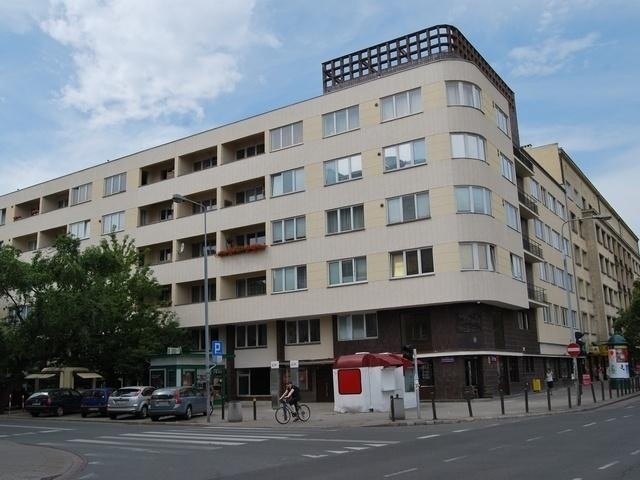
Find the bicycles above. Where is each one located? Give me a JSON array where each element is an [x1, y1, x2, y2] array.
[[274, 399, 312, 425]]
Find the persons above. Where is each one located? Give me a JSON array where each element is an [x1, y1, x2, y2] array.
[[279, 381, 301, 421], [544, 368, 555, 395]]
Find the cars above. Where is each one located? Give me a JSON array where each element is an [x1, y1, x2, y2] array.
[[20, 385, 217, 420]]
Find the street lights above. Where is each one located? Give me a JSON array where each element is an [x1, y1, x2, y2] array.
[[172, 192, 215, 425], [559, 214, 615, 341]]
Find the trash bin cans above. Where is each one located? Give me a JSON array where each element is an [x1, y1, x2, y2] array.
[[228, 401, 242, 422], [389, 398, 405, 420]]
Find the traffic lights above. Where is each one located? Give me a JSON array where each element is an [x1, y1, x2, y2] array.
[[402, 343, 414, 361], [574, 330, 585, 348]]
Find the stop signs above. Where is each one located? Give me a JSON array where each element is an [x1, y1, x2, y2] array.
[[566, 342, 582, 358]]
